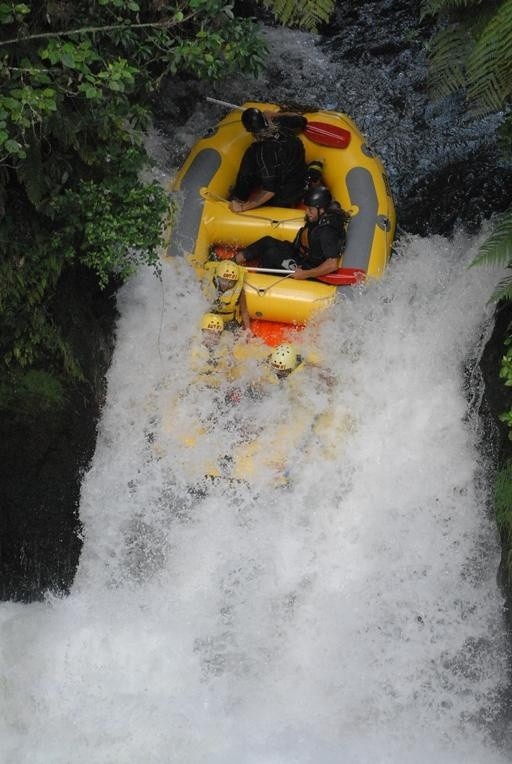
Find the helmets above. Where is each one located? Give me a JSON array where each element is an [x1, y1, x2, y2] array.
[[241, 107, 269, 132], [303, 186, 332, 209], [270, 344, 298, 372], [215, 260, 240, 281], [199, 313, 224, 331]]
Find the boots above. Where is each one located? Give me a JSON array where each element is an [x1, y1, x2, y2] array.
[[209, 250, 236, 262]]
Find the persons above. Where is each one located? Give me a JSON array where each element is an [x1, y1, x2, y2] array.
[[228, 105, 308, 212], [200, 259, 253, 339], [207, 185, 346, 280], [178, 313, 234, 412], [248, 342, 307, 402]]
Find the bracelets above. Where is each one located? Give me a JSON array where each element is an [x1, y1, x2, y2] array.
[[239, 201, 243, 212]]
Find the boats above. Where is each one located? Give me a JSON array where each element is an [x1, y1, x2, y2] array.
[[155, 98, 398, 494]]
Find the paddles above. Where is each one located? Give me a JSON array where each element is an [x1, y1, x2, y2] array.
[[199, 94, 350, 149], [205, 259, 365, 286]]
